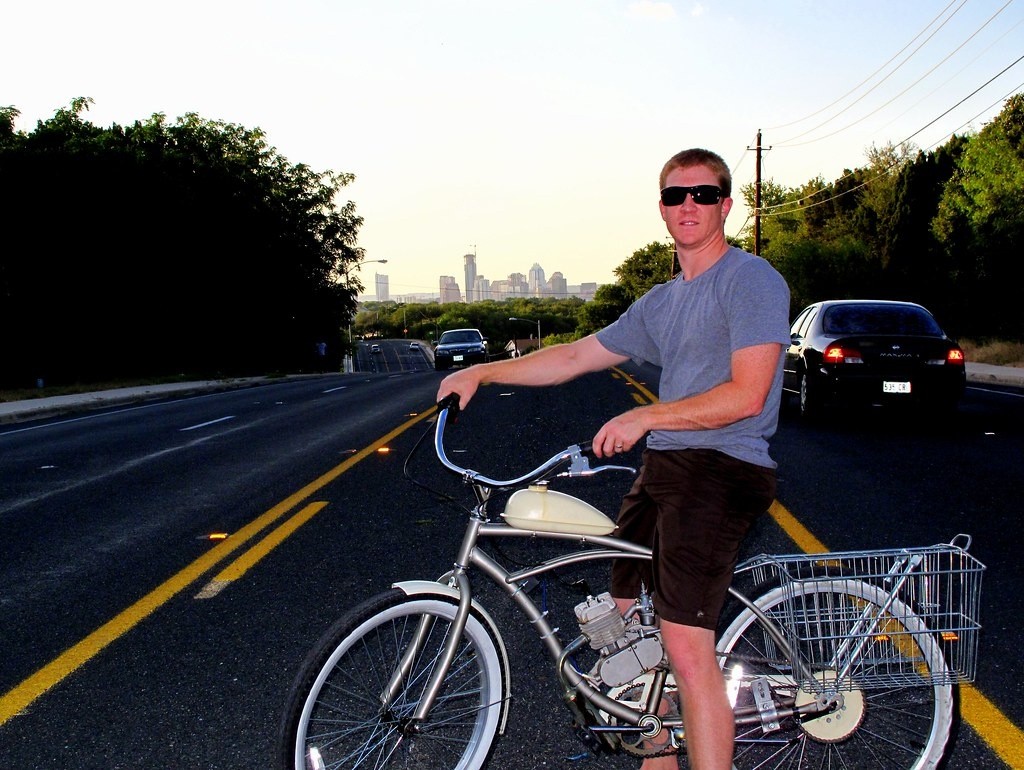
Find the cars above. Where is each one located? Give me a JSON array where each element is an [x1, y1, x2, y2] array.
[[781, 299, 967, 422], [370, 344, 381, 354], [433, 328, 490, 371], [410, 342, 419, 350]]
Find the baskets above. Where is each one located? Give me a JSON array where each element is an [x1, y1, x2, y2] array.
[[734, 531, 986, 694]]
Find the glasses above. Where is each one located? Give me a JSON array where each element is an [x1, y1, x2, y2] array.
[[661, 185, 721, 206]]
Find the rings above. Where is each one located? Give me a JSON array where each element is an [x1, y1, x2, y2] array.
[[614, 444, 622, 448]]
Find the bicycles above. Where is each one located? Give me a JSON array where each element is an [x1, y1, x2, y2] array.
[[278, 386, 987, 770]]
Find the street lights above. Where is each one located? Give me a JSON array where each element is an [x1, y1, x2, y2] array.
[[509, 317, 541, 351], [346, 259, 388, 355]]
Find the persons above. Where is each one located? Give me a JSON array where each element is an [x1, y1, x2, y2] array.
[[436, 149, 789, 770]]
